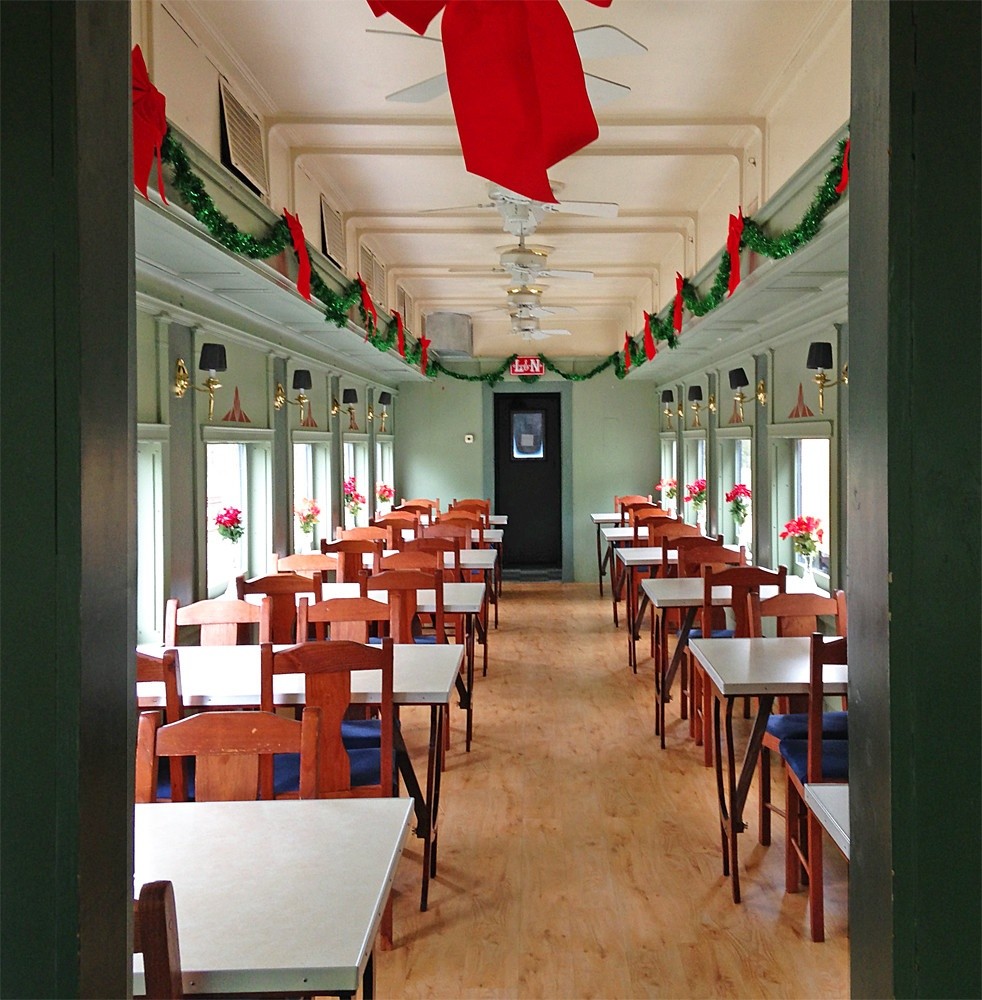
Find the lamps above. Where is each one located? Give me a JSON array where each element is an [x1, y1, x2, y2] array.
[[174, 342, 392, 420], [660, 342, 848, 428]]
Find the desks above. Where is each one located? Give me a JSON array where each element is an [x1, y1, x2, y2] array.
[[590, 513, 848, 904], [133, 516, 509, 1000]]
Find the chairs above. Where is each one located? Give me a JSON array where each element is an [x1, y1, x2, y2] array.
[[614, 494, 848, 944], [130, 497, 490, 1000]]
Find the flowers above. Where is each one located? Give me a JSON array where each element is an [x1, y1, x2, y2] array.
[[682, 479, 706, 509], [375, 483, 395, 503], [780, 515, 823, 555], [214, 506, 244, 544], [343, 477, 366, 514], [726, 484, 752, 527], [666, 480, 677, 498], [292, 498, 320, 533]]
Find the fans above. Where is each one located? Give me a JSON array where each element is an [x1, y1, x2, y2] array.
[[360, 24, 647, 340]]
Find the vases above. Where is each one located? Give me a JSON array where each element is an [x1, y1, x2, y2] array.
[[800, 554, 817, 593], [737, 525, 749, 557], [224, 545, 241, 599], [696, 510, 706, 535], [301, 535, 311, 555]]
